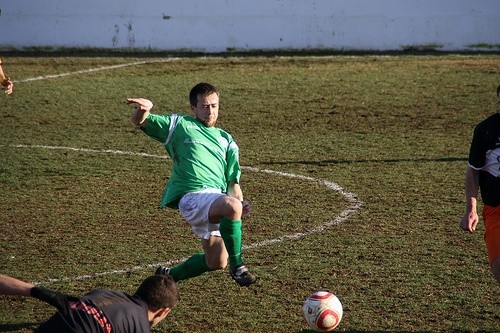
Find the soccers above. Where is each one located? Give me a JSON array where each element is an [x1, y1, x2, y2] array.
[[304, 291, 343, 332]]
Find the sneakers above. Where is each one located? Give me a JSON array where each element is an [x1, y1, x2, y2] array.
[[229, 265, 257, 287], [155, 266, 170, 275]]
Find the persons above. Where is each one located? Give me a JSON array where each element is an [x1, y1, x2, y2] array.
[[0, 59, 13, 95], [459, 82, 500, 288], [126, 82, 257, 287], [0, 271, 178, 333]]
